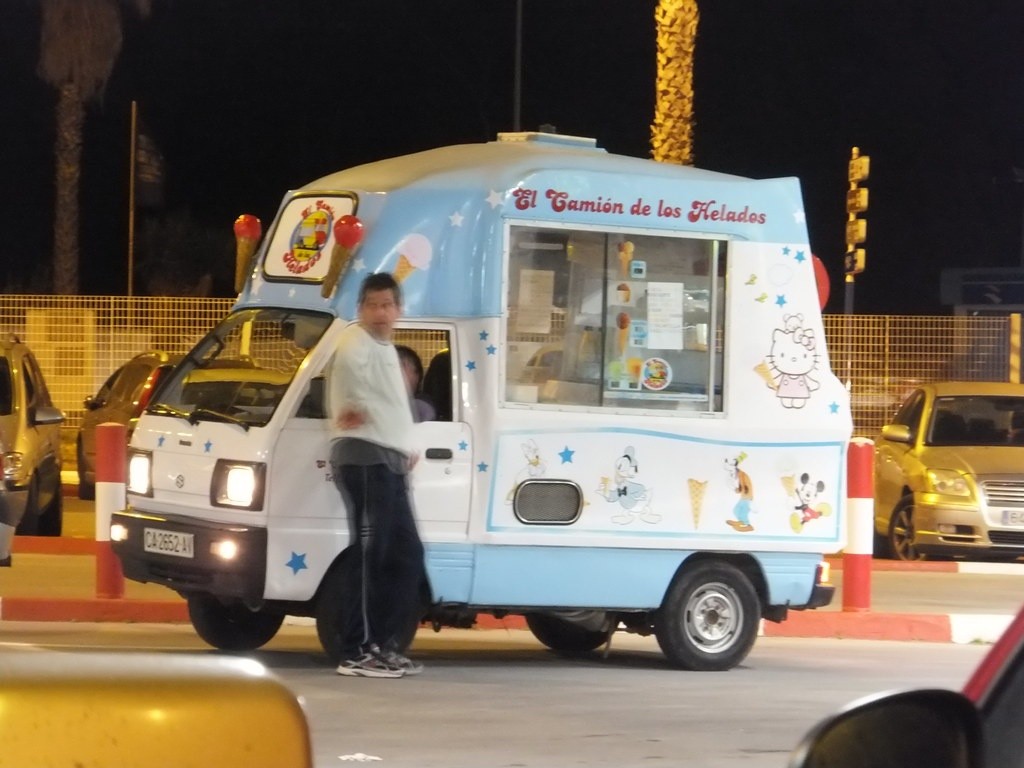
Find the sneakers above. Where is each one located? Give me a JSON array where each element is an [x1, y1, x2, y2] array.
[[336, 641, 405, 678], [381, 646, 424, 675]]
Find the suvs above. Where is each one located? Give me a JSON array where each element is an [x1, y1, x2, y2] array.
[[0, 333, 67, 537], [76, 350, 262, 500]]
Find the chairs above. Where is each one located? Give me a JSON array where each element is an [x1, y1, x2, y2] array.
[[934, 414, 966, 434], [422, 330, 453, 421]]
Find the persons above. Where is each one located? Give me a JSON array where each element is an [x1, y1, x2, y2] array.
[[324, 272, 430, 678], [393, 346, 437, 424]]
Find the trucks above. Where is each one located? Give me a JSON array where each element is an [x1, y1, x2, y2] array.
[[108, 131, 853, 672]]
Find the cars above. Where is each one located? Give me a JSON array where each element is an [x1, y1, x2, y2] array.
[[874, 381, 1024, 561], [789, 605, 1024, 768]]
